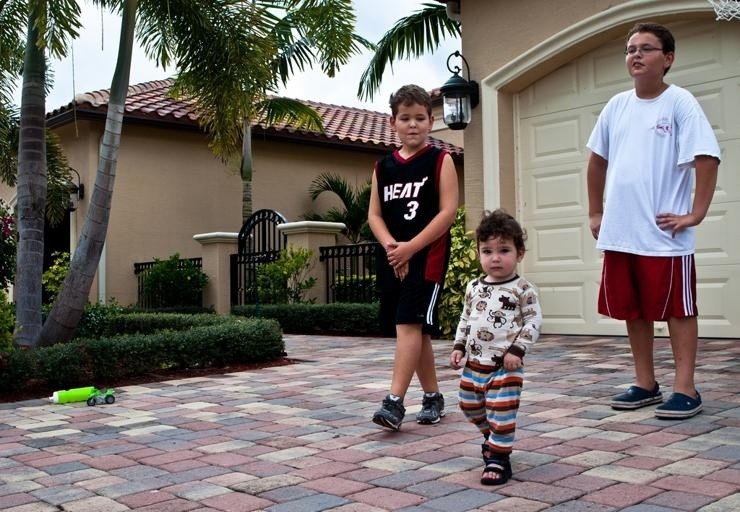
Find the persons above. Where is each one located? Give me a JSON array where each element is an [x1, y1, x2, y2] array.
[[365, 84, 461, 435], [582, 21, 725, 420], [449, 209, 544, 486]]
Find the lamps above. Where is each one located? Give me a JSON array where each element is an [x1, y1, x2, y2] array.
[[62, 167, 83, 212], [441, 52, 480, 130]]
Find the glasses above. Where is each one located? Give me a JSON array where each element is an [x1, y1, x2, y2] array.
[[624, 47, 663, 55]]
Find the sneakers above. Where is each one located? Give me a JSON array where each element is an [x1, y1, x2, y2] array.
[[416, 391, 445, 425], [610, 380, 662, 410], [656, 388, 703, 420], [374, 395, 406, 432]]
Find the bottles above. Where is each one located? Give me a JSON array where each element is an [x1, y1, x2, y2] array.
[[48, 386, 94, 403]]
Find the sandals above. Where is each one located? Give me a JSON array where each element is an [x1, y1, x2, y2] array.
[[481, 434, 512, 485]]
